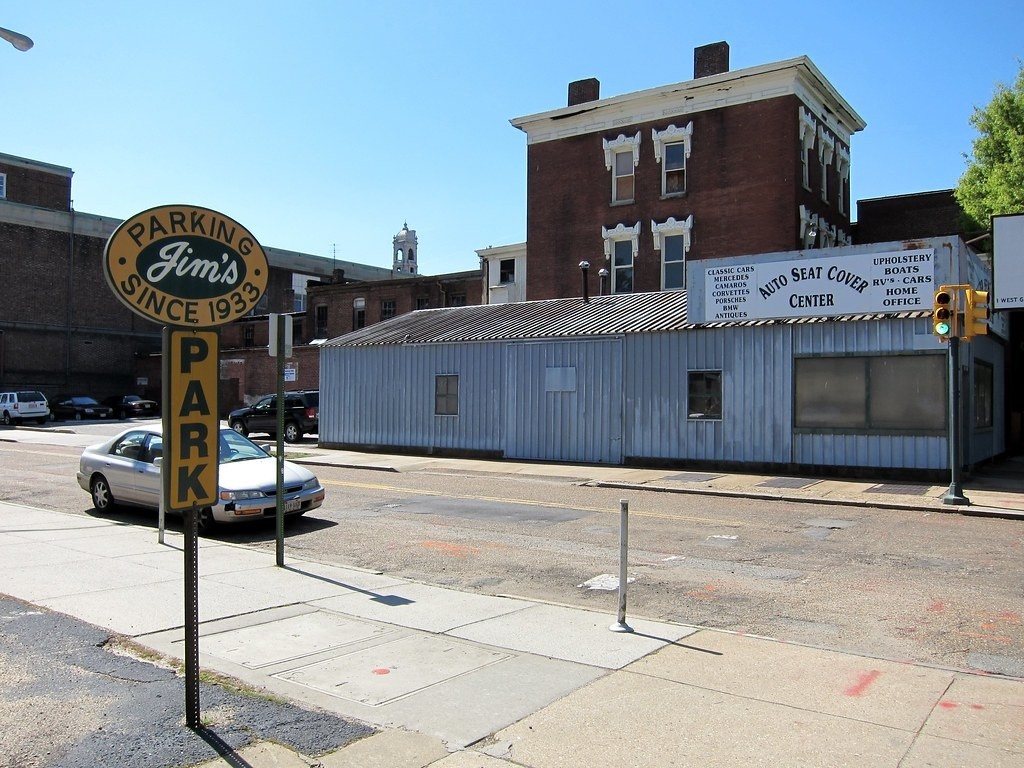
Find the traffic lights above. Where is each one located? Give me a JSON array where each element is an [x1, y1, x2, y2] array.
[[931, 284, 991, 344]]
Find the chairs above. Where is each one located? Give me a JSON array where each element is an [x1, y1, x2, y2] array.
[[121, 443, 162, 463]]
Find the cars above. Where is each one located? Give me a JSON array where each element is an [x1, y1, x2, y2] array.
[[0, 389, 159, 425], [73, 422, 327, 536], [227, 388, 320, 445]]
